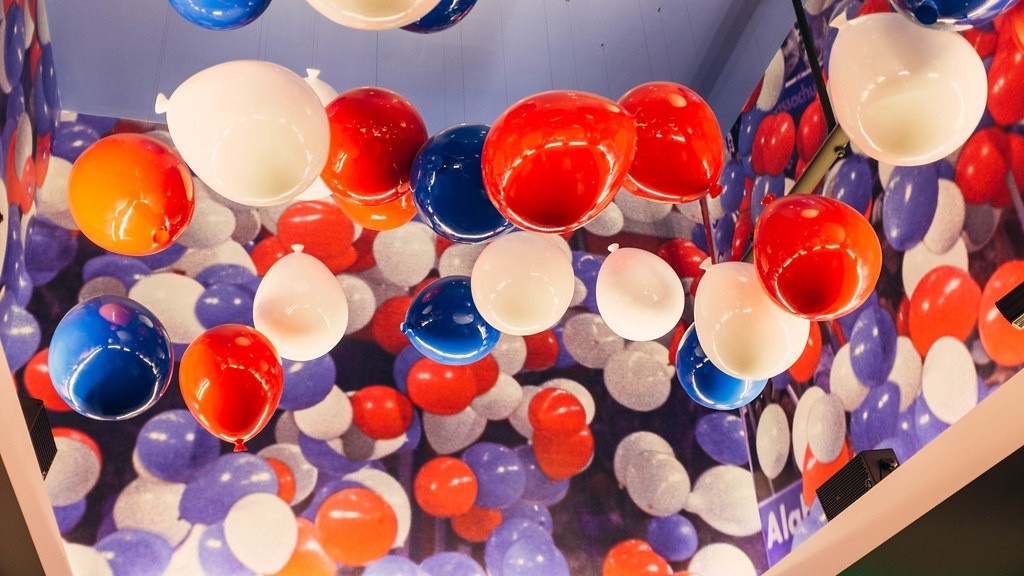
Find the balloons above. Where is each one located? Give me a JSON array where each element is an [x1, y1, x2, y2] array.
[[0, 0, 1024, 576]]
